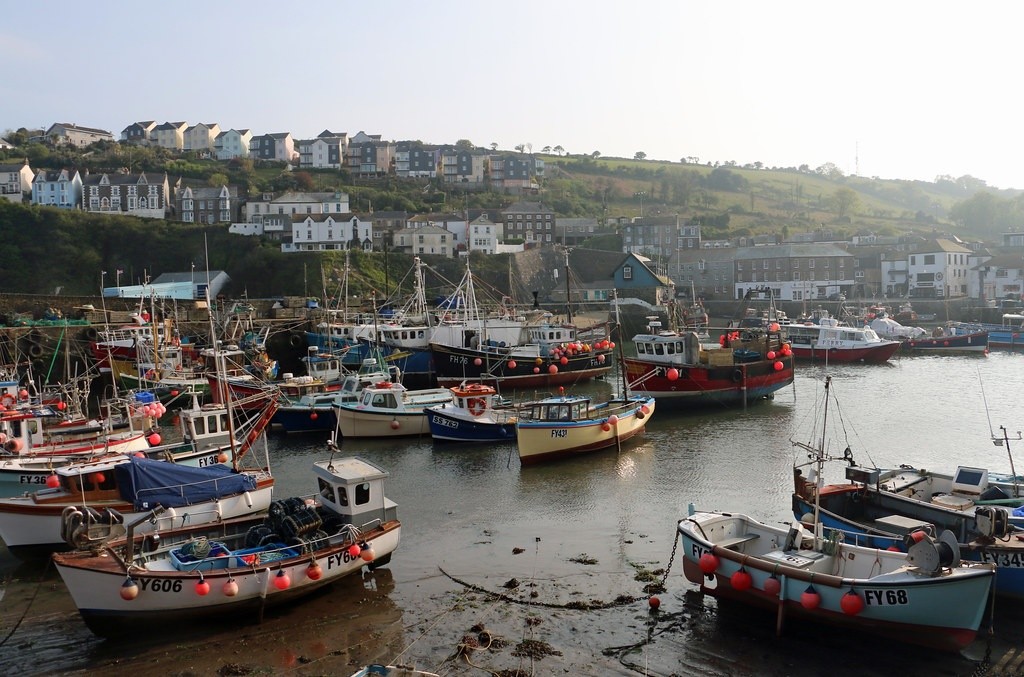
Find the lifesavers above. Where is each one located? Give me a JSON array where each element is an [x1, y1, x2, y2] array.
[[467, 397, 488, 417], [0, 393, 15, 410], [376, 381, 393, 389], [730, 369, 745, 384]]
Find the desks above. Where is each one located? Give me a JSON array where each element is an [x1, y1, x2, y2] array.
[[276, 382, 325, 399]]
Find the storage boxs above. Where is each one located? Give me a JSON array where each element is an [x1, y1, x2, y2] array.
[[234, 544, 299, 567], [169, 544, 232, 572]]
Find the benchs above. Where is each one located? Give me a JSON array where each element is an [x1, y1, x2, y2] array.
[[880, 472, 931, 501], [717, 533, 760, 548], [608, 398, 646, 414]]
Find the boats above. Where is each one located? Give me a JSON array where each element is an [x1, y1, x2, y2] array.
[[678, 501, 997, 649], [873, 328, 988, 353], [616, 322, 799, 410], [945, 314, 1023, 347], [51, 455, 402, 639], [786, 374, 1024, 600], [766, 317, 903, 364]]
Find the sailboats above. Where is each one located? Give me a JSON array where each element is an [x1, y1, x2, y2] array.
[[2, 256, 939, 537], [513, 287, 658, 468]]
